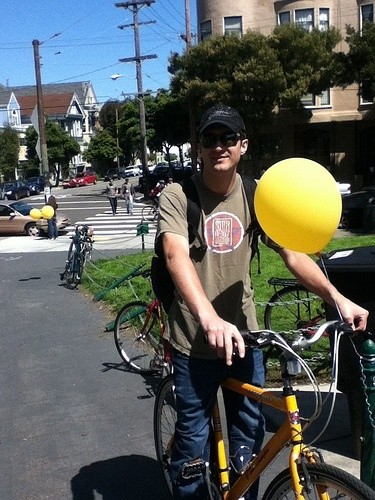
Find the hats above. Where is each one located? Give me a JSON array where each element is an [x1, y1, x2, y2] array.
[[198, 105, 247, 135]]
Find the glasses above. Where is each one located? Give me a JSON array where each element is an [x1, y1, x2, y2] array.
[[200, 132, 244, 149]]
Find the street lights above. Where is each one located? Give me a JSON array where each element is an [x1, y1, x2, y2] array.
[[109, 72, 137, 82]]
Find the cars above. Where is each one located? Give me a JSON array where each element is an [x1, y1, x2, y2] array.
[[143, 158, 192, 173], [0, 181, 30, 201], [125, 165, 140, 177], [104, 167, 126, 181], [25, 177, 46, 195], [69, 171, 97, 187], [0, 200, 70, 236]]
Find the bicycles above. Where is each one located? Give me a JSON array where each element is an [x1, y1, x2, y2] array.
[[153, 320, 374, 500], [112, 267, 168, 373], [61, 221, 95, 290], [141, 202, 159, 221], [263, 278, 338, 366]]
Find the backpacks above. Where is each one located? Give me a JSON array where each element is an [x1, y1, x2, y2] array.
[[150, 174, 261, 314]]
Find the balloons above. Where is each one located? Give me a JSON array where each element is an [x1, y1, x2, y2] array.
[[254, 156, 343, 256], [29, 208, 41, 219], [41, 205, 55, 220]]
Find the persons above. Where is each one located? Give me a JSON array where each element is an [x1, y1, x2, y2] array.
[[166, 177, 174, 185], [150, 108, 369, 500], [104, 179, 120, 215], [46, 195, 59, 241], [140, 179, 166, 208], [119, 178, 134, 214]]
